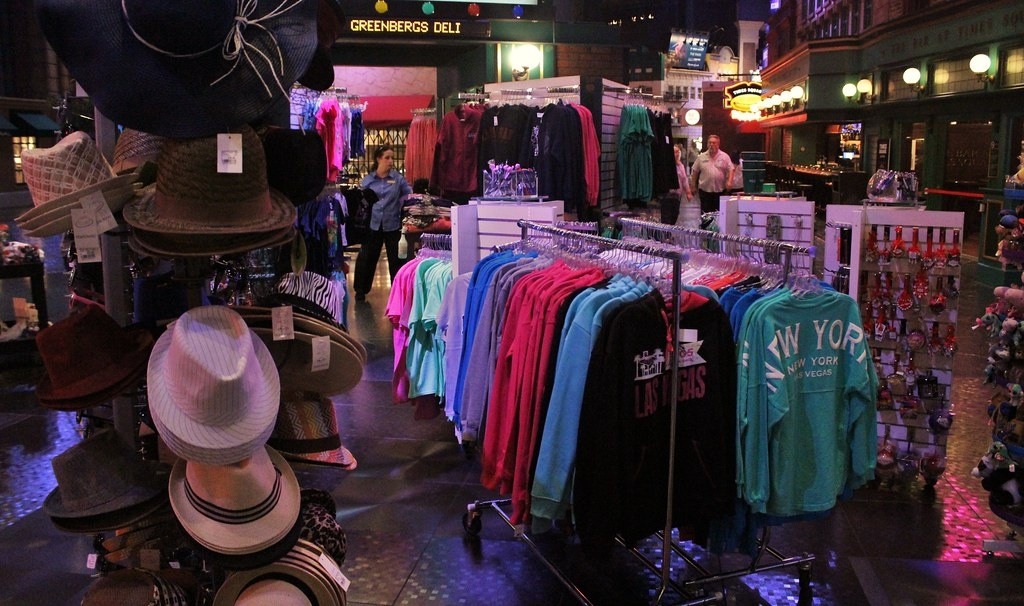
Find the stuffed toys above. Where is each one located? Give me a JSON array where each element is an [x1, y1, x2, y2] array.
[[970, 168, 1024, 509]]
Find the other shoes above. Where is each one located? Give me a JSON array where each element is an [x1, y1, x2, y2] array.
[[354, 288, 365, 300]]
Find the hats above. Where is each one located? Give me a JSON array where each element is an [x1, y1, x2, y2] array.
[[15, 0, 365, 605]]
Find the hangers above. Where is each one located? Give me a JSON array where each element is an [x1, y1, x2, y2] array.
[[453, 91, 492, 107], [523, 222, 822, 304], [419, 231, 452, 252], [292, 83, 360, 109], [495, 88, 531, 111], [647, 97, 671, 115], [620, 91, 646, 107], [539, 84, 577, 109], [414, 107, 438, 120]]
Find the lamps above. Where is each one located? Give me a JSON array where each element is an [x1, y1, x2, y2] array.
[[764, 97, 773, 117], [793, 87, 803, 109], [781, 91, 792, 114], [771, 93, 780, 115], [903, 67, 922, 98], [970, 54, 995, 85], [857, 79, 872, 104], [841, 83, 856, 104]]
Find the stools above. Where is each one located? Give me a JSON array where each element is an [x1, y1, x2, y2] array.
[[799, 185, 812, 198]]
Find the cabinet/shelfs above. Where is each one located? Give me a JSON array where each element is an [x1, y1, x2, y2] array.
[[0, 260, 50, 352]]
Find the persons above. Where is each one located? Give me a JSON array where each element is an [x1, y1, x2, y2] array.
[[691, 135, 734, 234], [728, 150, 747, 192], [353, 145, 415, 304], [661, 144, 692, 228]]
